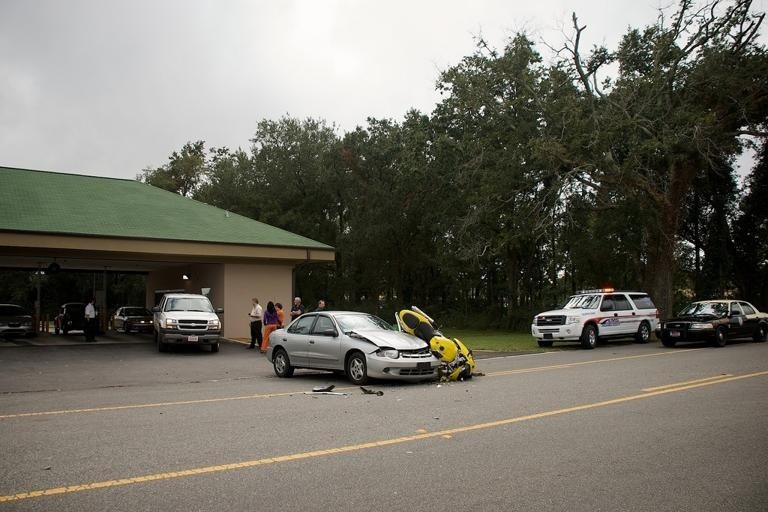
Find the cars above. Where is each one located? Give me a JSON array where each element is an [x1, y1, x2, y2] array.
[[0, 302, 34, 340], [108, 304, 155, 334], [262, 308, 440, 386], [54, 301, 87, 337], [655, 299, 767, 349]]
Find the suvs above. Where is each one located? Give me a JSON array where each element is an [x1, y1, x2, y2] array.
[[151, 292, 225, 354], [530, 287, 663, 350]]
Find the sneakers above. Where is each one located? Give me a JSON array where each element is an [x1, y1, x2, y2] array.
[[246, 347, 254, 349]]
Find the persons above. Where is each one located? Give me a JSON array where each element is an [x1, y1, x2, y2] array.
[[273, 302, 285, 329], [312, 299, 327, 312], [246, 297, 263, 349], [83, 297, 98, 342], [259, 300, 282, 355], [289, 296, 305, 322]]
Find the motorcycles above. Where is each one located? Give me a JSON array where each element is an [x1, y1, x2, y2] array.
[[393, 303, 480, 384]]
